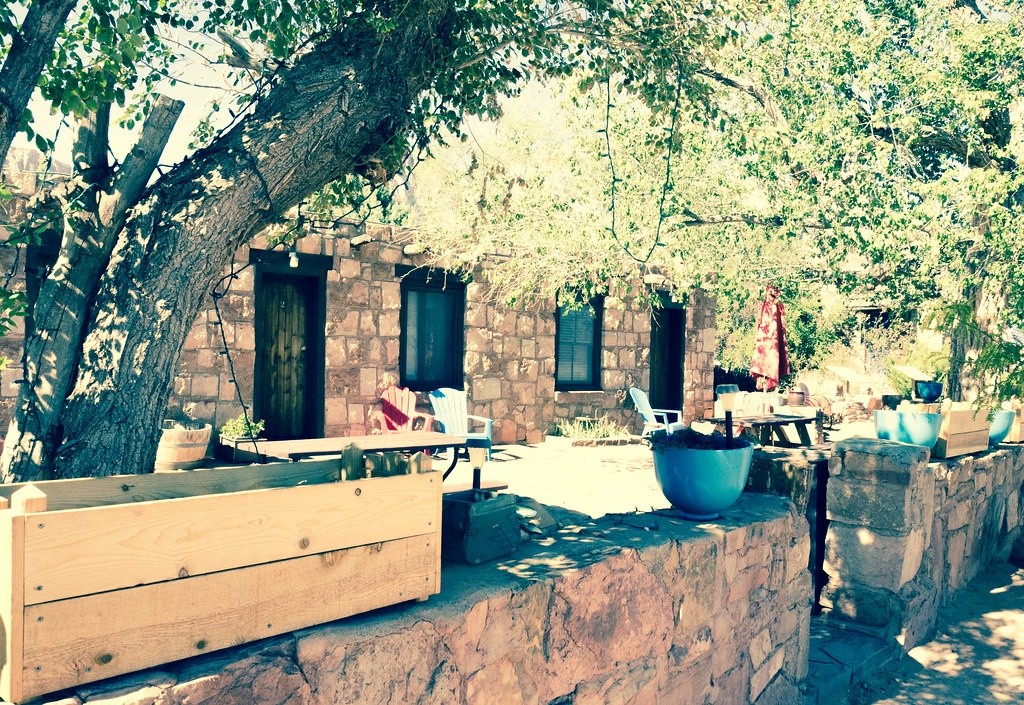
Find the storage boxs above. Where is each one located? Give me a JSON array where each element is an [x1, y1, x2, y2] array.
[[0, 442, 445, 705], [897, 398, 993, 457]]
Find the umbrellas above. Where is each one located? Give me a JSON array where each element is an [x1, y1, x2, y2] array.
[[749, 284, 790, 416]]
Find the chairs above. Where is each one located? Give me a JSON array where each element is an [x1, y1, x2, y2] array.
[[629, 388, 686, 437], [429, 387, 495, 462], [379, 385, 435, 455]]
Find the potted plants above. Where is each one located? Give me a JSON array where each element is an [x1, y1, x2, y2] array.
[[644, 427, 754, 519]]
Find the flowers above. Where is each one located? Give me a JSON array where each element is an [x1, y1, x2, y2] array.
[[219, 413, 266, 437]]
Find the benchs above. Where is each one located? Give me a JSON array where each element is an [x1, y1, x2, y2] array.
[[443, 478, 507, 496]]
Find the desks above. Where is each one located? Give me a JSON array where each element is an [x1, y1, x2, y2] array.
[[701, 413, 822, 447], [227, 430, 470, 480]]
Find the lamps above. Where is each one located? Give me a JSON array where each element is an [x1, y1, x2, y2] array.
[[645, 274, 666, 285]]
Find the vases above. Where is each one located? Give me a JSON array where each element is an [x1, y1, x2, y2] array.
[[155, 421, 213, 471], [873, 409, 944, 450], [989, 410, 1017, 447], [788, 391, 806, 405], [917, 383, 943, 402], [218, 434, 267, 444]]
